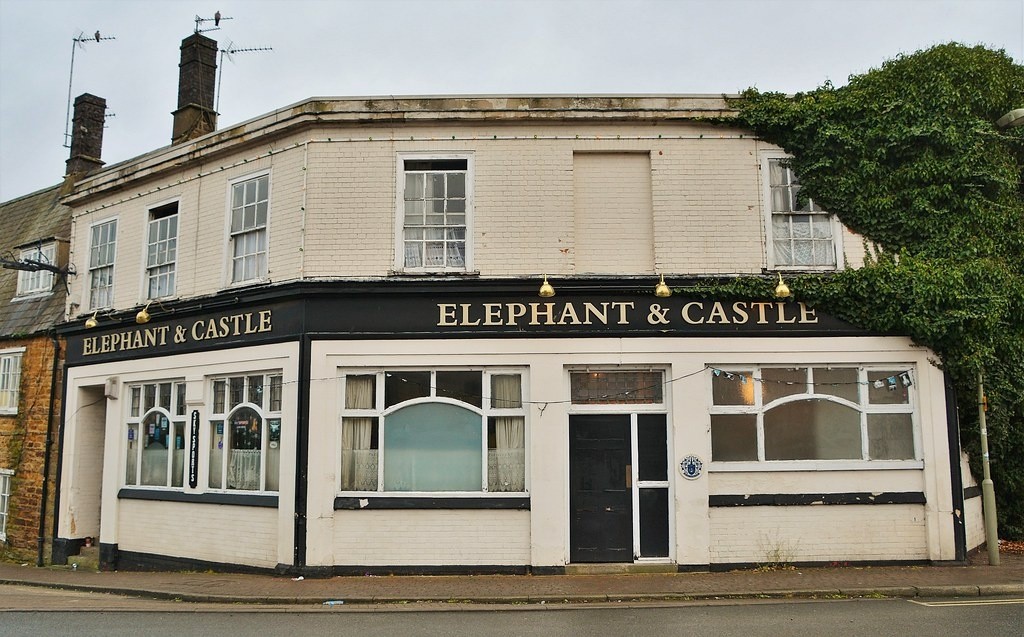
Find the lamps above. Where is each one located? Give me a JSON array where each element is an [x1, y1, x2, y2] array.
[[84, 309, 123, 329], [655, 274, 672, 297], [538, 274, 556, 297], [774, 272, 791, 298], [136, 299, 175, 323]]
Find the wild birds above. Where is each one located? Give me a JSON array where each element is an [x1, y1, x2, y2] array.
[[214, 10, 221, 27], [95, 30, 100, 42]]
